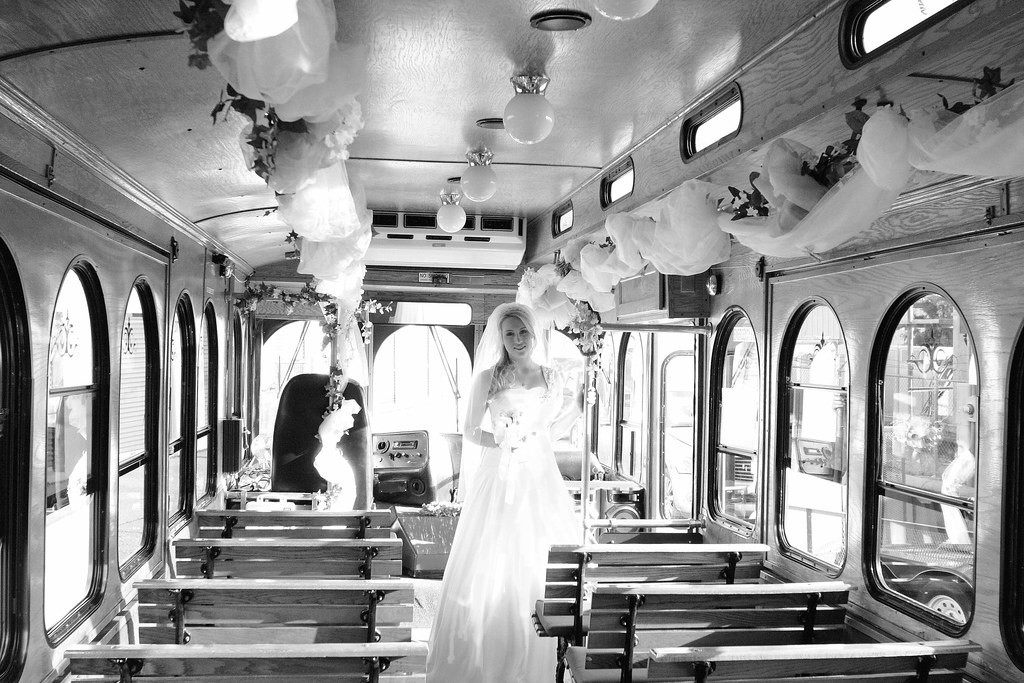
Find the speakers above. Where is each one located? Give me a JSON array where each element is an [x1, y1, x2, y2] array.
[[599, 480, 646, 532]]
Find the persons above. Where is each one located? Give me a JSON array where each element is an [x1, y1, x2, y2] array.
[[426, 304, 596, 683]]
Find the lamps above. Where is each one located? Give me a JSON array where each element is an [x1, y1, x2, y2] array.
[[503, 73, 554, 145], [437, 183, 466, 233], [460, 142, 498, 202], [595, 0, 658, 20]]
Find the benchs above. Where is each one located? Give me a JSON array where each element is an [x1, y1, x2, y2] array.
[[60, 490, 1024, 683]]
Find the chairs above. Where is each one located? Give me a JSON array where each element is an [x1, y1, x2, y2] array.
[[270, 373, 371, 510]]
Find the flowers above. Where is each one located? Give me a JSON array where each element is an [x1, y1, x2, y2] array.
[[726, 186, 766, 220], [574, 299, 611, 404], [800, 141, 857, 189], [181, 6, 221, 70], [255, 287, 393, 415], [322, 99, 366, 162], [248, 122, 278, 179]]
